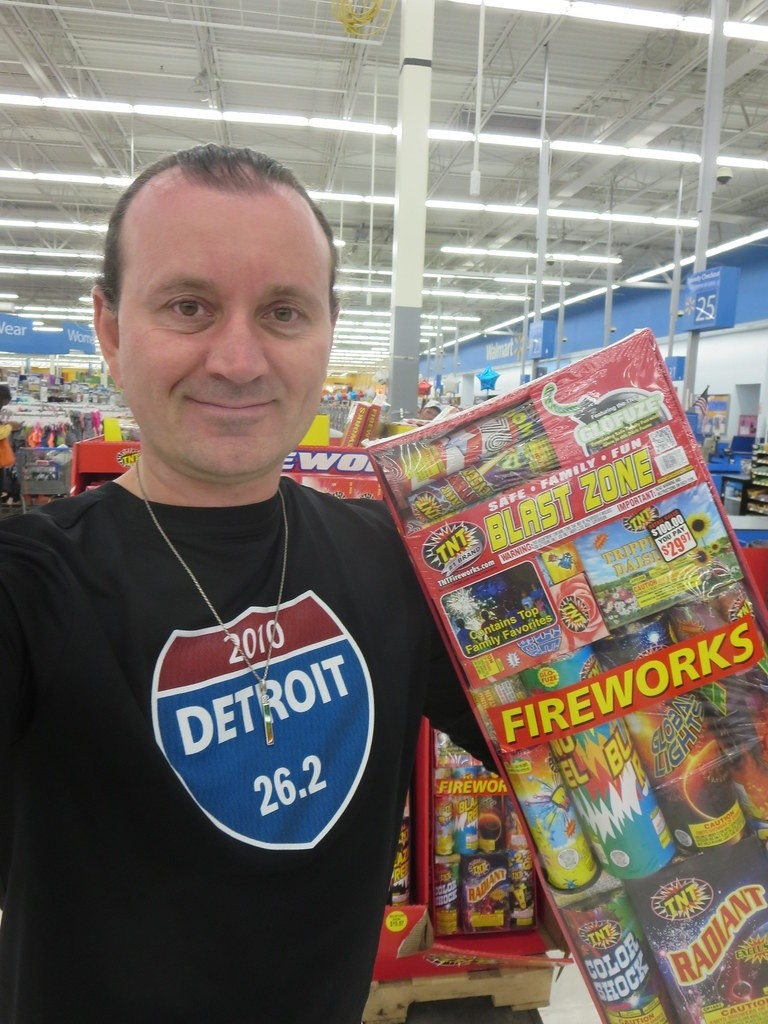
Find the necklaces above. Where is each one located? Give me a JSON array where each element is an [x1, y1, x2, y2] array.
[[135, 461, 288, 748]]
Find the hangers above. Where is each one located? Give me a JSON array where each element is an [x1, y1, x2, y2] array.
[[0, 402, 93, 429]]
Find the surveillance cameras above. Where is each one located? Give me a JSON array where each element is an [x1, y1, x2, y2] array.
[[717, 168, 734, 186], [546, 256, 555, 266]]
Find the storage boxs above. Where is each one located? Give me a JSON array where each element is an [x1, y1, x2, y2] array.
[[344, 328, 768, 1024]]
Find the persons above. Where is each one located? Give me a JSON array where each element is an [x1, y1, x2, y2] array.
[[0, 145, 501, 1024], [0, 384, 18, 467]]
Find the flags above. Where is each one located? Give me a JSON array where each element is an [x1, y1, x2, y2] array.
[[694, 389, 709, 414]]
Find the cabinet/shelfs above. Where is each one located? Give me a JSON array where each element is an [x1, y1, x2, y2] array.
[[741, 440, 768, 519]]
